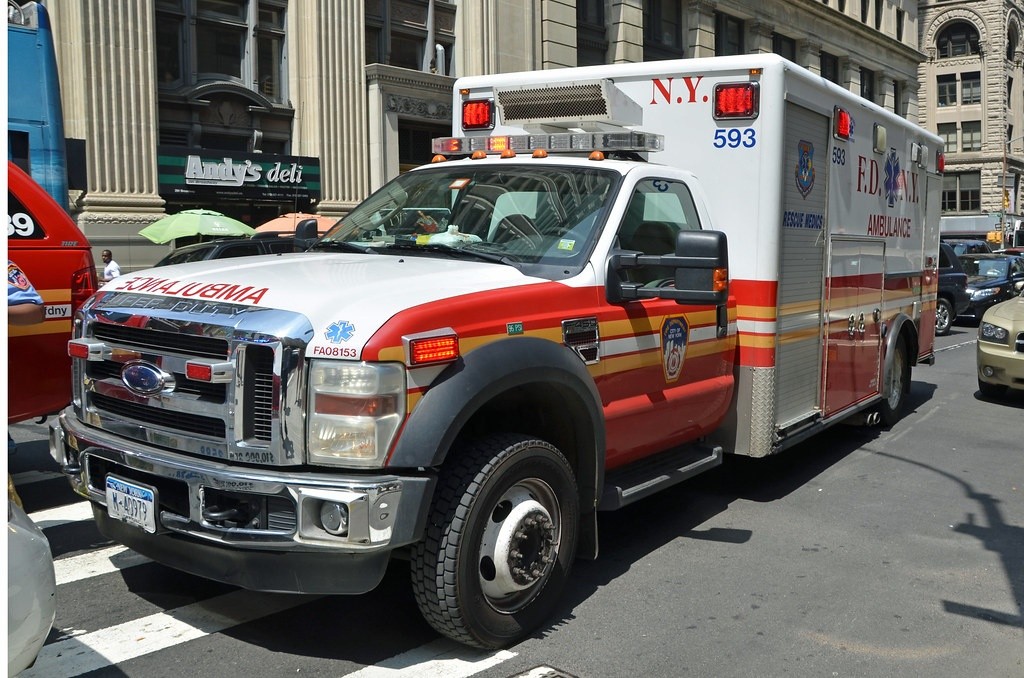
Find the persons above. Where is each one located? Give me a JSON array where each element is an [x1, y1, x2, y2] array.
[[415, 210, 437, 231], [98, 250, 120, 284]]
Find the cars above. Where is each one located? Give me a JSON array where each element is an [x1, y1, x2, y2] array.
[[8, 477, 58, 678], [957, 254, 1024, 322], [977, 280, 1024, 393], [942, 239, 992, 255], [992, 247, 1024, 256]]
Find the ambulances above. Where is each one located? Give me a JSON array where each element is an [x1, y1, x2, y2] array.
[[47, 52, 945, 650]]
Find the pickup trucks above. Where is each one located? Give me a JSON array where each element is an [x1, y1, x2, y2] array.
[[8, 161, 100, 426]]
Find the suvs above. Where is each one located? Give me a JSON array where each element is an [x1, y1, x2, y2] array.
[[934, 240, 970, 337]]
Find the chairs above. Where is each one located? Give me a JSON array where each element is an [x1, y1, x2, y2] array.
[[628, 221, 677, 284], [492, 214, 543, 253]]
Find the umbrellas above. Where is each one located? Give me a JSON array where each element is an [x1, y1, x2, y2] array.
[[138, 208, 256, 245], [254, 212, 360, 238]]
